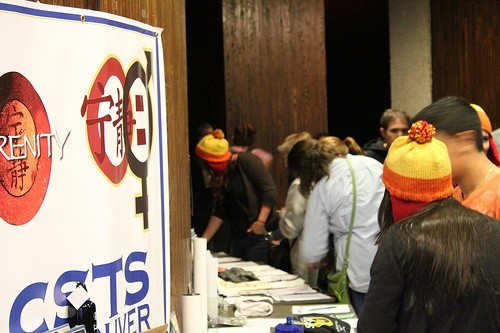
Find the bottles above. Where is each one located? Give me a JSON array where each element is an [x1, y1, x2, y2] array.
[[76, 282, 97, 333], [274, 316, 304, 333]]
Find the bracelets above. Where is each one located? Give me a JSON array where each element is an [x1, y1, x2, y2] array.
[[258, 220, 265, 224]]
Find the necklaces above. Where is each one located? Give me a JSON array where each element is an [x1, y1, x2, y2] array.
[[471, 164, 495, 193]]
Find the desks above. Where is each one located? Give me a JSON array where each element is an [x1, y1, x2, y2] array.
[[207, 251, 359, 333]]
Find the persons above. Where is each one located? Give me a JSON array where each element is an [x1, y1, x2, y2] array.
[[276, 131, 385, 316], [195, 123, 278, 267], [357, 96, 500, 333]]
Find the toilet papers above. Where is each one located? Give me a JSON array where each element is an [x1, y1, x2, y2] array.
[[193, 237, 209, 332], [181, 292, 203, 332]]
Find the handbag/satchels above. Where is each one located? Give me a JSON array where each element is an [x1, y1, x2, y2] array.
[[327, 267, 351, 304]]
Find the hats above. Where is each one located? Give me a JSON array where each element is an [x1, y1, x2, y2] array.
[[470, 103, 493, 138], [195, 129, 231, 162], [381, 120, 455, 201]]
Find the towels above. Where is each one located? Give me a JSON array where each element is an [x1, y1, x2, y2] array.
[[234, 293, 273, 317]]
